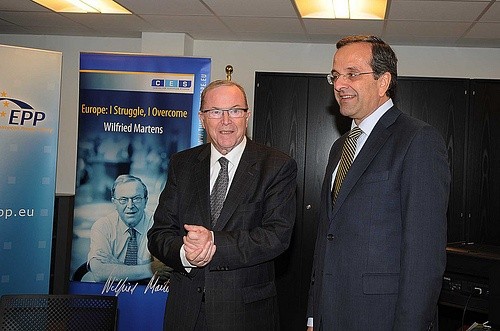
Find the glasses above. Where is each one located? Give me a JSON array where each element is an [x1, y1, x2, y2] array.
[[326, 71, 377, 85], [114, 196, 145, 205], [202, 108, 247, 119]]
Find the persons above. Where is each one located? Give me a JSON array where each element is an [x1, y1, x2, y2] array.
[[87, 175, 173, 282], [147, 80, 297, 331], [304, 34, 451, 331]]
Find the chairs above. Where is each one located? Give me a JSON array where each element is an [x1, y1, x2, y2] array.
[[0, 295, 119, 331]]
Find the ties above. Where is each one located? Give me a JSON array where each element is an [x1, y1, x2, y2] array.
[[124, 228, 138, 266], [332, 127, 363, 210], [209, 157, 229, 230]]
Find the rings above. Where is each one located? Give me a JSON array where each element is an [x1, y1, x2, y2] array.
[[204, 260, 208, 262]]
